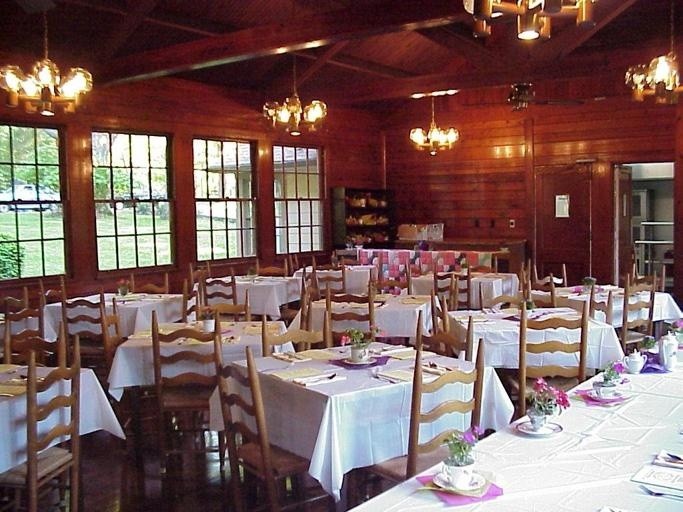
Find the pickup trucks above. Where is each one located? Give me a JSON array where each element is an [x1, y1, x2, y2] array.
[[0, 183, 60, 215]]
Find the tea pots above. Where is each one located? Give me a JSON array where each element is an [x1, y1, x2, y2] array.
[[659, 331, 679, 370], [623, 349, 647, 374]]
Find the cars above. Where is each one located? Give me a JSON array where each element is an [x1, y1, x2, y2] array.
[[110, 192, 163, 210]]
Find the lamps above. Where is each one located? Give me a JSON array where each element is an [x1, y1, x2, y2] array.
[[462, 0, 596, 41], [1, 12, 93, 116], [409, 98, 460, 156], [625, 0, 683, 105], [263, 55, 327, 136]]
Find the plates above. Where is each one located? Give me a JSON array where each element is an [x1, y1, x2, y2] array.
[[344, 357, 378, 365], [515, 420, 563, 436], [586, 390, 626, 402], [432, 472, 486, 490]]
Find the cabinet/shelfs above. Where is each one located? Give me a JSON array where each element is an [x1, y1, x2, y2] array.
[[631, 189, 653, 277], [331, 186, 394, 249]]
